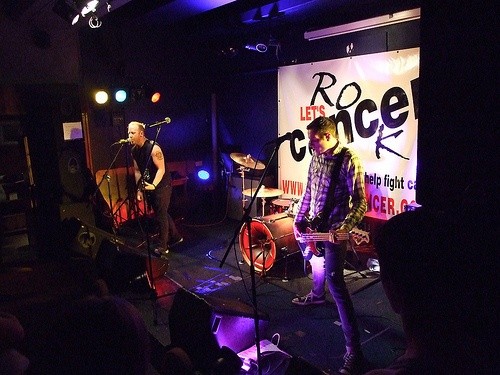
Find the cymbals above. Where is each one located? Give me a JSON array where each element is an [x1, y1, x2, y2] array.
[[273, 198, 299, 206], [242, 186, 285, 198], [230, 152, 265, 170]]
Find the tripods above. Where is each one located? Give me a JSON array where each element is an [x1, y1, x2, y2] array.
[[249, 230, 294, 288]]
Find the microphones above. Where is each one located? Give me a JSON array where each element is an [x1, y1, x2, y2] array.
[[112, 137, 132, 147], [149, 116, 172, 128], [269, 132, 293, 145]]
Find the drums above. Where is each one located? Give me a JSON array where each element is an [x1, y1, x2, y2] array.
[[239, 210, 302, 272]]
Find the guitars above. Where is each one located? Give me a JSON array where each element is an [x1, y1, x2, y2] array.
[[294, 212, 371, 261]]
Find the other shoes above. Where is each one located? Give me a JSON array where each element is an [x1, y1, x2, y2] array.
[[292, 289, 325, 305], [165, 236, 183, 254], [338, 352, 363, 374]]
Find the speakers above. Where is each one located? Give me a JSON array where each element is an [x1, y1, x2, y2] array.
[[64, 216, 115, 258], [93, 237, 170, 289], [168, 285, 270, 354], [226, 173, 272, 220], [51, 201, 95, 233]]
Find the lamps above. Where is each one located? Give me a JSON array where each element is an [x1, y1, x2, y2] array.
[[305, 6, 421, 43], [68, 0, 99, 16], [51, 0, 80, 27], [241, 31, 283, 55]]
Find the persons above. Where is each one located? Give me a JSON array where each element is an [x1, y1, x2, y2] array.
[[292, 117, 363, 374], [119, 119, 184, 256], [365, 205, 500, 375], [0, 257, 243, 375]]
